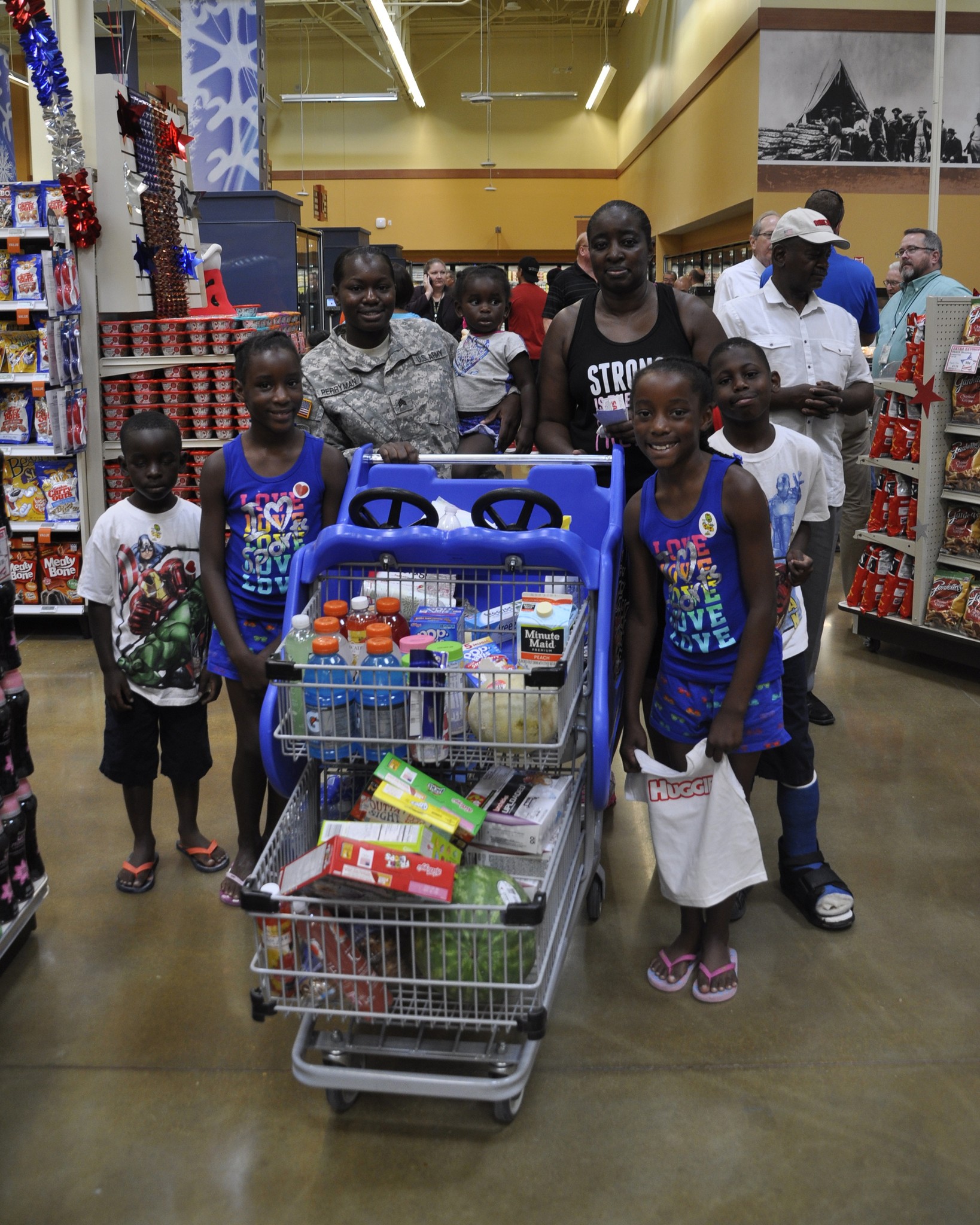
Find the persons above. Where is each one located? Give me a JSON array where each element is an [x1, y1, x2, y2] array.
[[199, 327, 351, 908], [77, 408, 231, 894], [611, 355, 788, 1005], [705, 336, 857, 934], [295, 184, 975, 724]]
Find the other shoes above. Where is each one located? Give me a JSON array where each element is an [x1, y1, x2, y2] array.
[[777, 835, 855, 929], [730, 886, 752, 921]]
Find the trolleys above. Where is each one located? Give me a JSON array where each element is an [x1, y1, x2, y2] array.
[[238, 442, 628, 1121]]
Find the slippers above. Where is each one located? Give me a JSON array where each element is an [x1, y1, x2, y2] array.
[[176, 839, 229, 873], [692, 948, 738, 1002], [648, 948, 702, 992], [220, 864, 247, 906], [117, 852, 159, 893]]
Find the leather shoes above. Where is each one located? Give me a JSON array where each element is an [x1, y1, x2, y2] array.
[[806, 691, 835, 724]]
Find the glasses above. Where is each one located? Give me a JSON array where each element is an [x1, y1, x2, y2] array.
[[894, 247, 934, 258], [757, 231, 772, 239], [883, 280, 902, 287]]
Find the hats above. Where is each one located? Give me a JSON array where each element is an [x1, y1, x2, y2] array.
[[771, 207, 850, 250], [519, 257, 539, 282]]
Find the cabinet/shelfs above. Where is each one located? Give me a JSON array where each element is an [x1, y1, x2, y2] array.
[[96, 349, 239, 531], [837, 295, 980, 668], [0, 218, 94, 623]]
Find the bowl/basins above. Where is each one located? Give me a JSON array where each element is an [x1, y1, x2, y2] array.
[[99, 304, 301, 506]]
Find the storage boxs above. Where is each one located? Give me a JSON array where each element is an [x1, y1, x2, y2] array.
[[274, 573, 576, 913]]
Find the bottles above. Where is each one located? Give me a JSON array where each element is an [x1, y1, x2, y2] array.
[[0, 451, 45, 922], [257, 879, 406, 1011], [286, 596, 466, 765]]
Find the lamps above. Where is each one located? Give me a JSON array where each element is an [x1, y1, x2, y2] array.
[[278, 0, 651, 113]]
